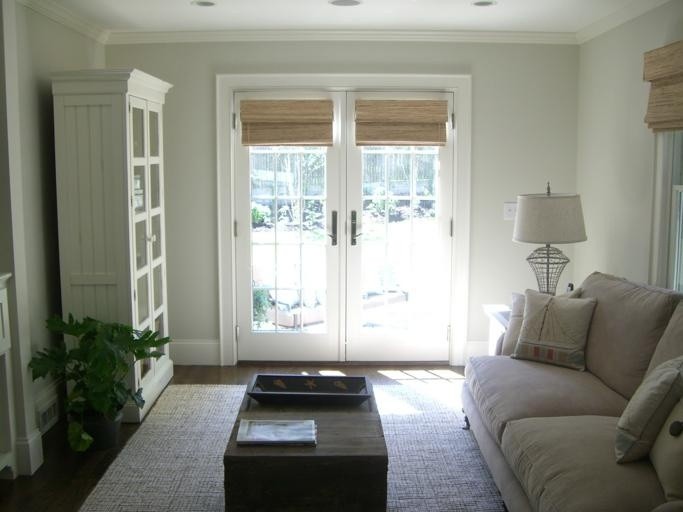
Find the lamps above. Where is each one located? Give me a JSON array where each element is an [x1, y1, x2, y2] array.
[[513, 183, 588, 295]]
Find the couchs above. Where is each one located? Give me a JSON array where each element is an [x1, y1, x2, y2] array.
[[461, 271, 682, 512]]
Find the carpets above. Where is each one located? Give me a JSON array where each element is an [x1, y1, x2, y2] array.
[[79, 377, 508, 511]]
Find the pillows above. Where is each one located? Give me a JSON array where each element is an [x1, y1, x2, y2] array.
[[614, 358, 681, 465], [513, 292, 597, 371]]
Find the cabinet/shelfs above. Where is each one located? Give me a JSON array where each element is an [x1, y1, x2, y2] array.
[[53, 81, 174, 421]]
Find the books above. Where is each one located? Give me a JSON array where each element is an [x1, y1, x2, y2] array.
[[236, 418, 317, 446]]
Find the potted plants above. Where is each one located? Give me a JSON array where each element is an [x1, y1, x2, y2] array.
[[27, 313, 172, 472]]
